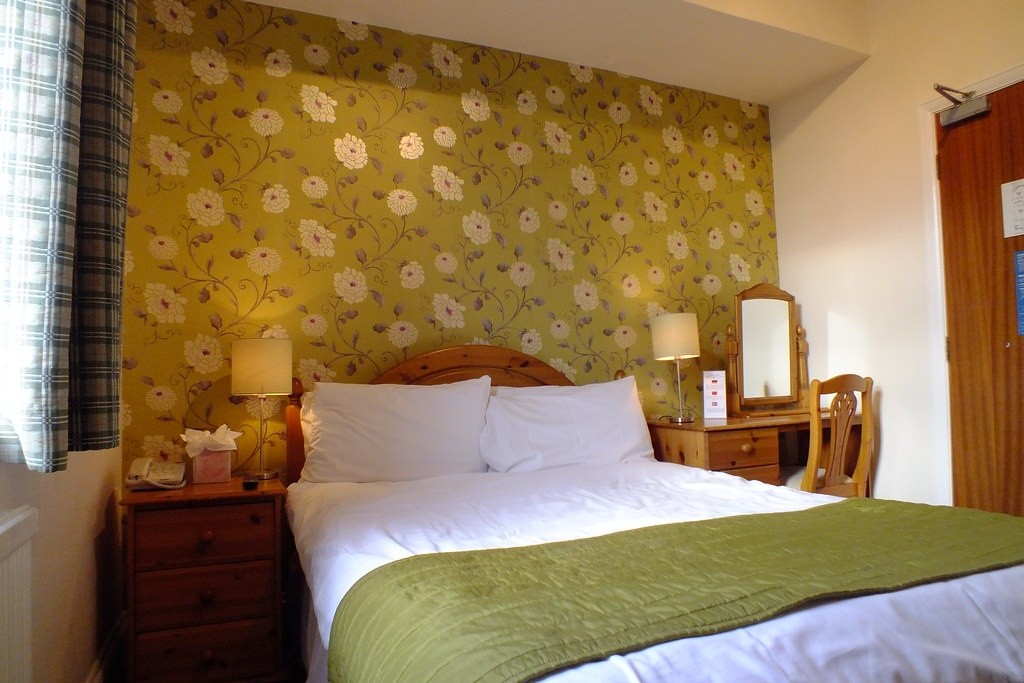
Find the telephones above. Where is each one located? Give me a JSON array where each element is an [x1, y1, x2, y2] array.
[[124, 457, 186, 490]]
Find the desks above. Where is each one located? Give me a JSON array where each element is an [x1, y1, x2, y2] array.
[[646, 410, 873, 499]]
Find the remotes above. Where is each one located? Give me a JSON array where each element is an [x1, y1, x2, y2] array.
[[243, 472, 259, 489]]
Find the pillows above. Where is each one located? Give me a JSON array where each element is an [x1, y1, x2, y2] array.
[[297, 374, 492, 484], [479, 375, 655, 474]]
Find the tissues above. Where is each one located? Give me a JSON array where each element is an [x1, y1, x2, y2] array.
[[178, 423, 244, 484]]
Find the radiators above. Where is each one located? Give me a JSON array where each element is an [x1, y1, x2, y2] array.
[[0, 501, 40, 682]]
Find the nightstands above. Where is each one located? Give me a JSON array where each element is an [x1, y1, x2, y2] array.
[[119, 475, 290, 683]]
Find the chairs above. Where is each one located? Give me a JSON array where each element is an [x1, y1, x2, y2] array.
[[779, 374, 874, 499]]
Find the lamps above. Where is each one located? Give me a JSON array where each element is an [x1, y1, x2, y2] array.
[[650, 312, 701, 424], [231, 337, 294, 480]]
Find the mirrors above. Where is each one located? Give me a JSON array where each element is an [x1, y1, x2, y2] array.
[[741, 298, 792, 399]]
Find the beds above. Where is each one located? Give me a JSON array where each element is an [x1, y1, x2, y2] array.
[[283, 457, 1024, 683]]
